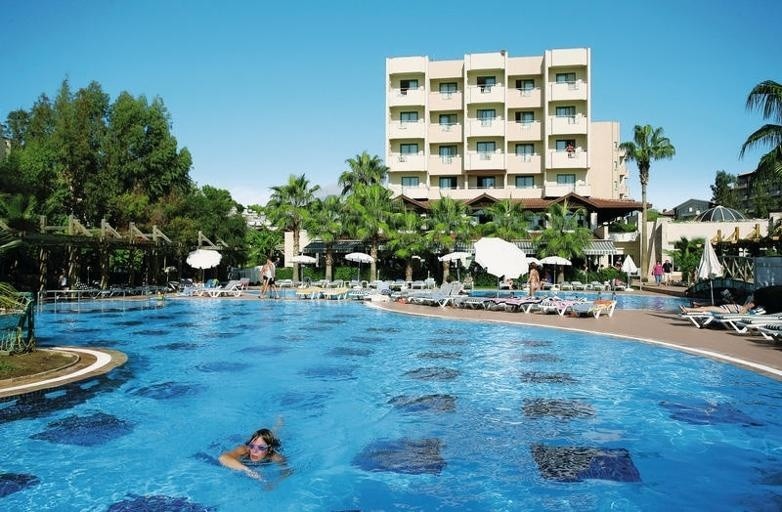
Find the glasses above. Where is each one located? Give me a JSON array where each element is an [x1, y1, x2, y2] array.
[[248, 444, 271, 450]]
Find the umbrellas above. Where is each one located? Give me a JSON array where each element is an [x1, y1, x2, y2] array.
[[621, 254, 637, 288], [287, 252, 317, 286], [474, 237, 543, 279], [439, 252, 472, 281], [693, 205, 747, 221], [697, 237, 724, 305], [186, 249, 222, 283], [540, 256, 572, 284], [345, 253, 374, 282]]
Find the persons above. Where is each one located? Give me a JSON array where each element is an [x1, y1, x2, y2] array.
[[58, 270, 70, 296], [565, 141, 575, 158], [499, 274, 514, 290], [527, 262, 541, 298], [652, 260, 673, 287], [433, 276, 452, 293], [258, 258, 280, 300], [542, 269, 552, 283], [218, 428, 295, 491], [678, 301, 757, 314]]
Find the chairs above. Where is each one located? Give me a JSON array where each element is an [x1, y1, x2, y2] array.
[[676, 294, 782, 341], [273, 278, 631, 321], [189, 278, 252, 298]]
